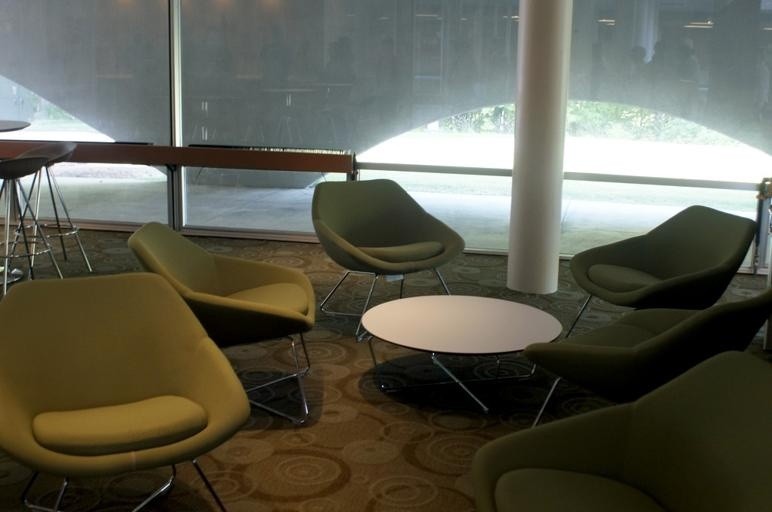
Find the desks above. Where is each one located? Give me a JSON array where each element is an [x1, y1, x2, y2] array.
[[0, 120, 30, 284], [186, 83, 355, 191]]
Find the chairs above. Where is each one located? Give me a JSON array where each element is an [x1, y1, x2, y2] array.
[[0, 277, 254, 512], [472, 352, 772, 512], [560, 206, 760, 340], [311, 178, 464, 344], [532, 289, 772, 423], [127, 221, 315, 428]]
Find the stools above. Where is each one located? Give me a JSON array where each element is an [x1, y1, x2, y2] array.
[[0, 156, 64, 297], [16, 141, 92, 279]]
